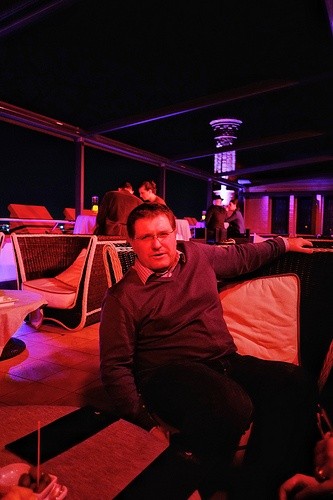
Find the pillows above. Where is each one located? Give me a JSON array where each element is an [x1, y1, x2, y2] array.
[[57, 249, 87, 286], [216, 273, 301, 370]]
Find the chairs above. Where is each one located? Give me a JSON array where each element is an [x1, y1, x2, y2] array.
[[11, 233, 121, 330]]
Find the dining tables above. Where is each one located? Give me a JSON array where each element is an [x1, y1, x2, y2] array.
[[1, 406, 201, 499], [75, 215, 191, 240], [0, 289, 46, 361], [104, 242, 333, 499]]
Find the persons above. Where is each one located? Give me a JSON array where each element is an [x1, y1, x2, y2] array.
[[204, 194, 228, 228], [99, 201, 328, 500], [278, 431, 333, 500], [226, 198, 247, 237], [94, 182, 144, 236], [139, 181, 168, 207]]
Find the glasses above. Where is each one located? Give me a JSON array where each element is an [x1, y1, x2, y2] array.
[[133, 230, 174, 242]]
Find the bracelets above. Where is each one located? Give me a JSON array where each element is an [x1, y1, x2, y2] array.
[[279, 236, 290, 253]]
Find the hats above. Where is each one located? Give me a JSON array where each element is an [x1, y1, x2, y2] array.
[[213, 195, 224, 201]]
